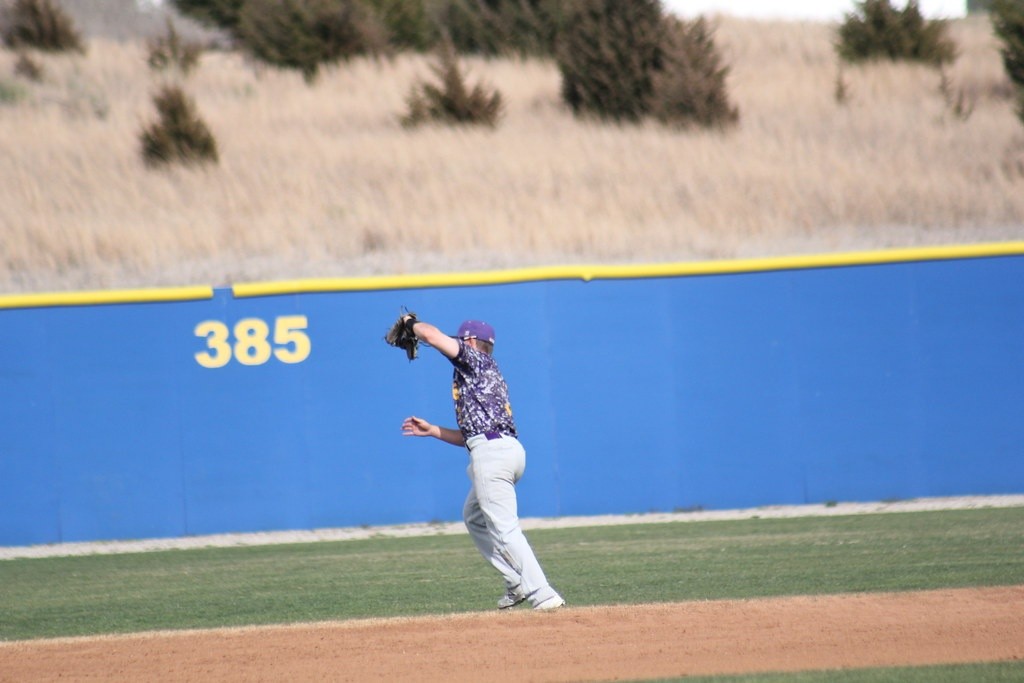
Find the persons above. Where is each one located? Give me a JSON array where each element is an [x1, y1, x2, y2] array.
[[385, 311, 566, 609]]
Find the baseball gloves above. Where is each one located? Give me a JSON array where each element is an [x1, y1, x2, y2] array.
[[385, 313, 417, 360]]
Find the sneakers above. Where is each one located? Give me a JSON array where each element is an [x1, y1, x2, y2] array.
[[497, 583, 525, 609], [534, 594, 565, 610]]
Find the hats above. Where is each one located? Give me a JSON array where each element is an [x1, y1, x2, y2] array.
[[449, 320, 495, 345]]
